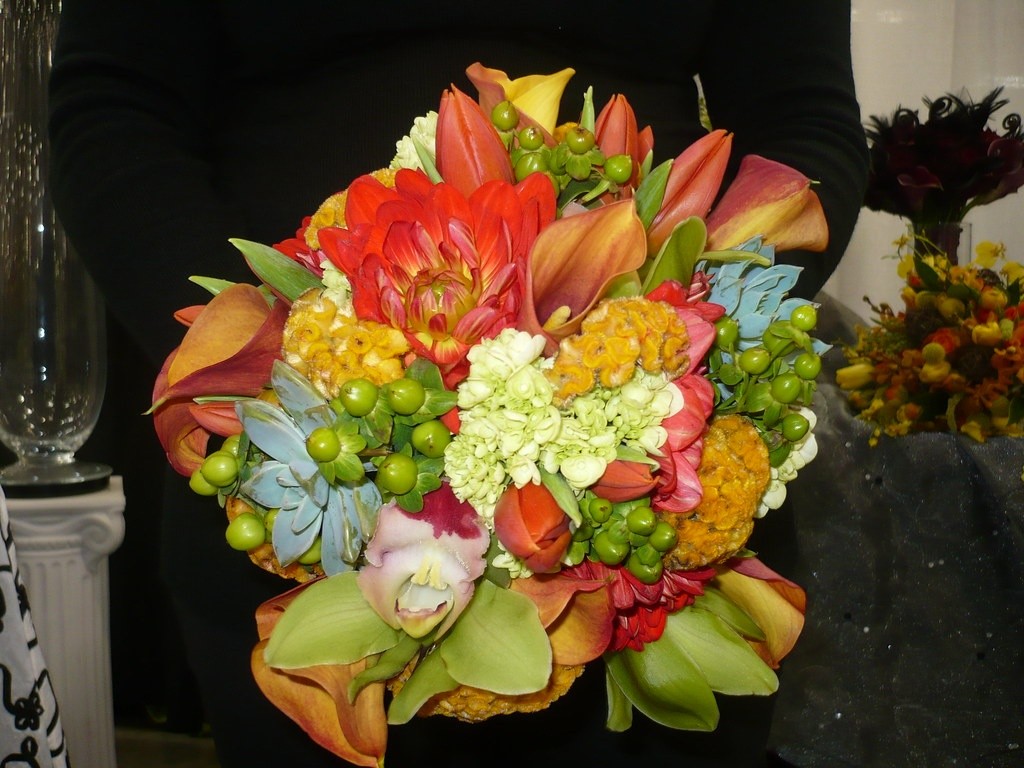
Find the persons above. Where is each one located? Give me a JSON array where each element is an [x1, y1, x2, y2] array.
[[37, 0, 873, 768]]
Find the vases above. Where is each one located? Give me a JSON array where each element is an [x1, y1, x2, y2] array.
[[0, 0, 113, 501], [905, 222, 973, 267]]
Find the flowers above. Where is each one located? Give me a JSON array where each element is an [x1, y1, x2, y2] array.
[[831, 222, 1024, 450], [859, 84, 1024, 266], [149, 61, 837, 768]]
